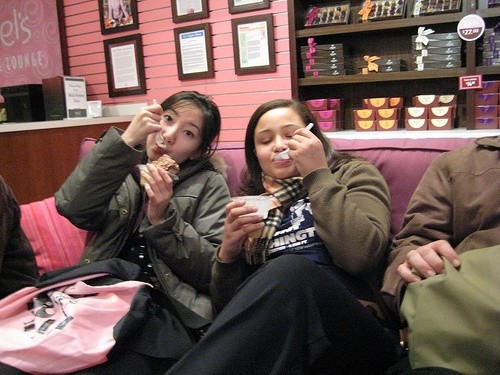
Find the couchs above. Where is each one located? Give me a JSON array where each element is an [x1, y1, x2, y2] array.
[[77, 130, 481, 236]]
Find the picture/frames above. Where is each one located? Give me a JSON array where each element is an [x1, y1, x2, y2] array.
[[231, 13, 276, 76], [102, 34, 147, 98], [171, 0, 209, 22], [228, 0, 270, 13], [97, 0, 141, 34], [173, 23, 214, 81]]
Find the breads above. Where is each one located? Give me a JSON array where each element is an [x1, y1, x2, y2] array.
[[148, 153, 180, 175]]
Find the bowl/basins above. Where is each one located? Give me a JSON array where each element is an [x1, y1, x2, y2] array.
[[136, 164, 179, 186], [229, 196, 281, 219]]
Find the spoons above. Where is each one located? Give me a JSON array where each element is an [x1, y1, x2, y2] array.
[[152, 99, 166, 149], [274, 123, 314, 160]]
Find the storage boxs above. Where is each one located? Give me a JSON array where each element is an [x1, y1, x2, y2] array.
[[298, 26, 500, 132], [409, 0, 463, 19], [300, 4, 351, 28], [357, 0, 407, 23]]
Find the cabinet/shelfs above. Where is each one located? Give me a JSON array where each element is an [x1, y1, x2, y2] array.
[[288, 0, 500, 137]]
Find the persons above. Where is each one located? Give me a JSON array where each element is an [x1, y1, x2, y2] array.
[[165, 100, 393, 375], [381, 134, 500, 375], [54, 90, 230, 375]]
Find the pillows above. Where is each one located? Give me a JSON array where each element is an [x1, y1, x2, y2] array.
[[18, 198, 90, 273]]
